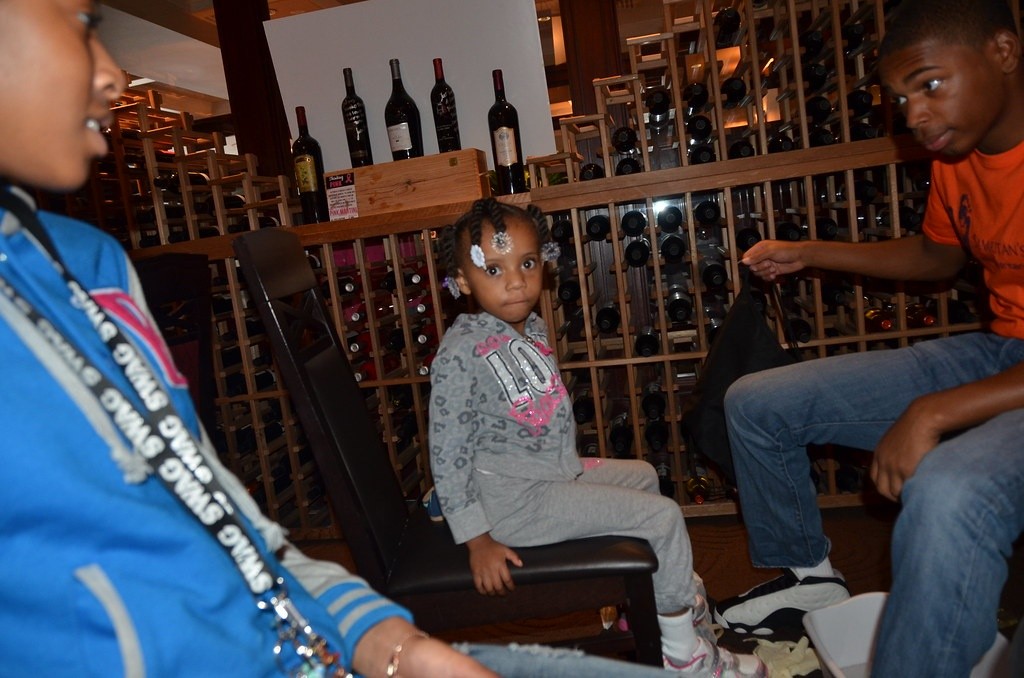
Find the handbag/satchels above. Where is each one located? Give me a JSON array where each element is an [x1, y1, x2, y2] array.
[[681, 290, 803, 471]]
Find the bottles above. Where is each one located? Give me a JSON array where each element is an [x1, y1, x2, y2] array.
[[488, 69, 526, 195], [93, 127, 280, 251], [342, 67, 373, 169], [292, 106, 329, 223], [384, 59, 425, 161], [430, 58, 462, 153], [208, 265, 477, 506], [549, 7, 990, 507]]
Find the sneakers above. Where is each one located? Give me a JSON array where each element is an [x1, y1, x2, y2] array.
[[715, 573, 852, 640], [662, 596, 769, 678]]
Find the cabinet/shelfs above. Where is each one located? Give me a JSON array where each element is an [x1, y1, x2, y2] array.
[[83, 0, 1024, 549]]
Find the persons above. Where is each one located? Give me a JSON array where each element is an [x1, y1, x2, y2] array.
[[715, 1, 1024, 678], [430, 197, 768, 678], [0, 0, 721, 678]]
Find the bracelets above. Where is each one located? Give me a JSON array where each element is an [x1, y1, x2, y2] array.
[[387, 630, 432, 678]]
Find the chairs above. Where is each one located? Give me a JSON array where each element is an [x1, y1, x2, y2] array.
[[132, 251, 214, 457], [228, 227, 668, 665]]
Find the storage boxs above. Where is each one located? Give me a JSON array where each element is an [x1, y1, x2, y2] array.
[[321, 145, 491, 222]]
